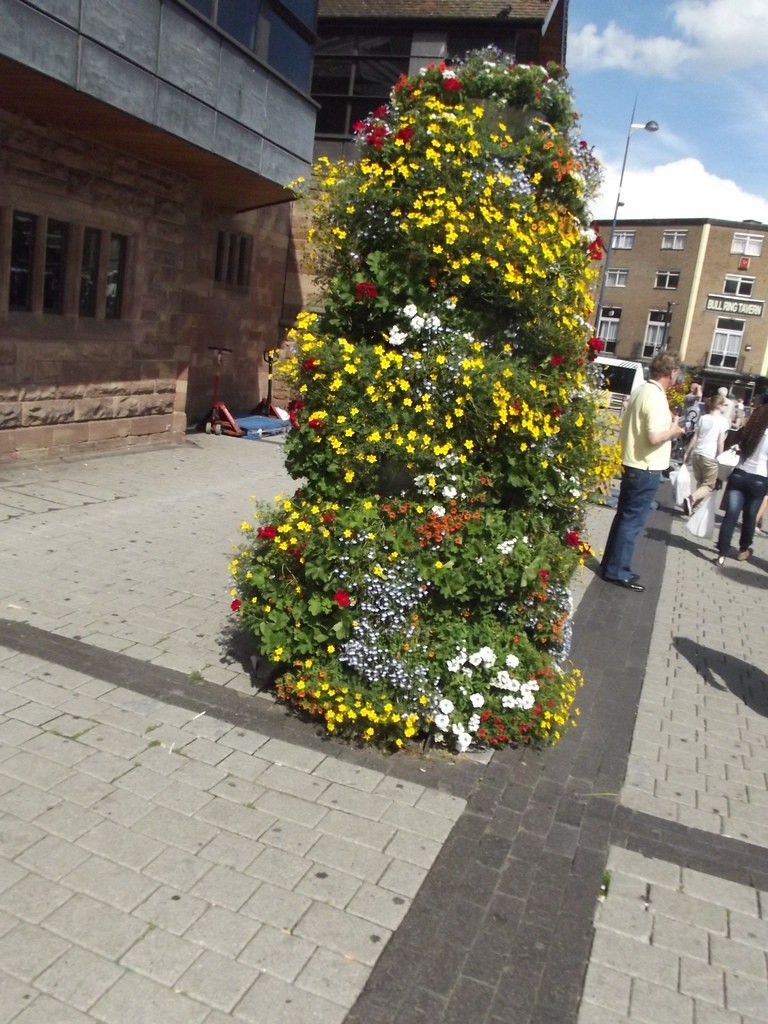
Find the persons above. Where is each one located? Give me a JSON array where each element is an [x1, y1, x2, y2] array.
[[598, 352, 682, 592], [671, 380, 768, 567]]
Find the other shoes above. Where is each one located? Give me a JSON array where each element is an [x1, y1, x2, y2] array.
[[737, 547, 754, 561], [714, 557, 725, 569], [684, 496, 694, 516]]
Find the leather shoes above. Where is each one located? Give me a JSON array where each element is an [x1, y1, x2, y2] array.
[[616, 580, 645, 592], [630, 575, 641, 582]]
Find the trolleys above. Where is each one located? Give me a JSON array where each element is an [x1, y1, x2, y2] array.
[[207, 346, 293, 436]]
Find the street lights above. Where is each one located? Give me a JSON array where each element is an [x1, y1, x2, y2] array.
[[593, 120, 660, 338]]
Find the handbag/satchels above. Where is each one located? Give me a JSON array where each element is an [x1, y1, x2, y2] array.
[[686, 488, 718, 540], [669, 464, 690, 506], [715, 441, 742, 481]]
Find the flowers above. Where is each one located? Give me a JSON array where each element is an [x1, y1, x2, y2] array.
[[232, 43, 627, 755]]
